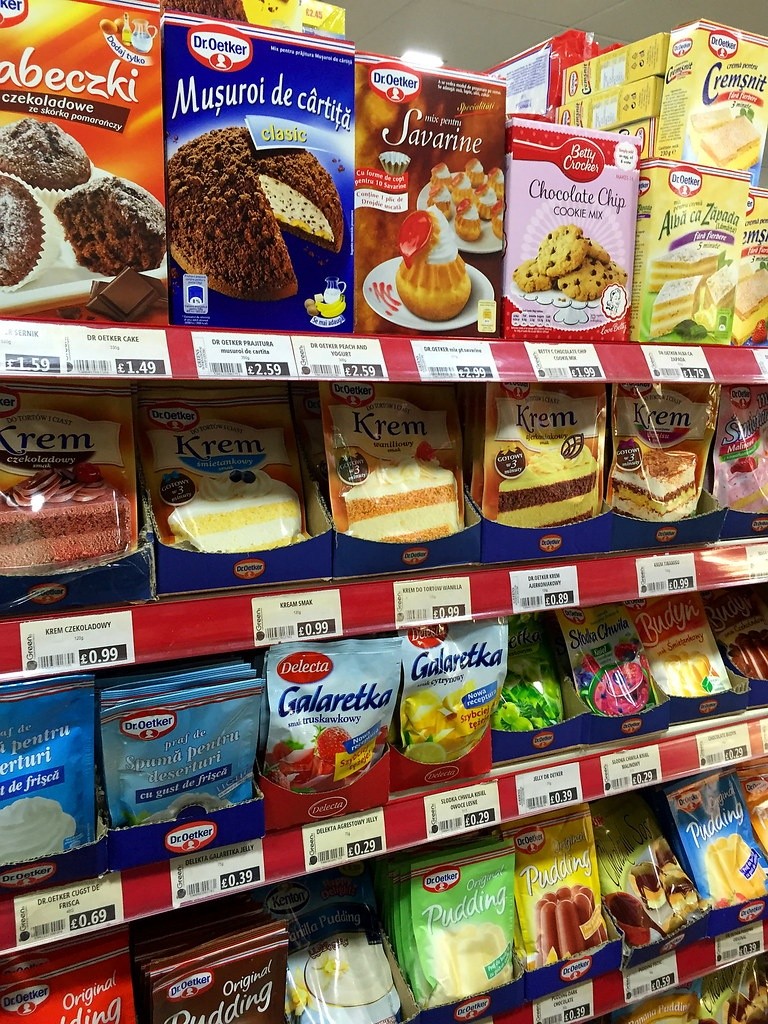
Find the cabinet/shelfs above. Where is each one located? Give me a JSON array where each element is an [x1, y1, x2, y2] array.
[[0, 321, 768, 1024]]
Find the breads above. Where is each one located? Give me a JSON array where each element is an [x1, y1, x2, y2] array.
[[646, 247, 768, 347]]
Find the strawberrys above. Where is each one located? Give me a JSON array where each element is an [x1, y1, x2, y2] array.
[[318, 725, 354, 763], [752, 319, 768, 343]]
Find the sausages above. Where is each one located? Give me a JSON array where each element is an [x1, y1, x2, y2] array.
[[535, 883, 608, 971]]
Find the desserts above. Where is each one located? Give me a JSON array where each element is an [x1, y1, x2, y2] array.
[[341, 445, 461, 544], [494, 440, 598, 528], [167, 127, 345, 302], [1, 462, 132, 569], [609, 449, 697, 521], [396, 159, 503, 321], [166, 470, 301, 553]]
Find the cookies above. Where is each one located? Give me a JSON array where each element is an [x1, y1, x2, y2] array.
[[516, 224, 629, 302]]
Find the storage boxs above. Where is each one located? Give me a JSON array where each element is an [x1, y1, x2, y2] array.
[[0, 0, 767, 345]]
